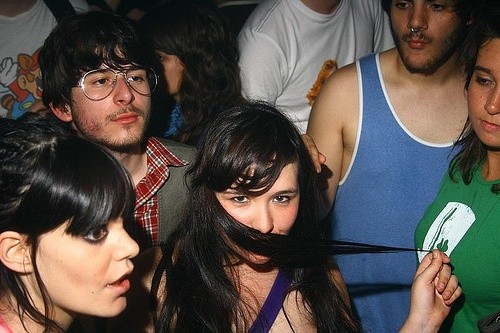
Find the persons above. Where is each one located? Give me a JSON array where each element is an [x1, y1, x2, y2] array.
[[0, 0, 500, 333], [0, 111, 139, 333]]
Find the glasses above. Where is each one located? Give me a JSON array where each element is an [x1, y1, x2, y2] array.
[[72, 66, 159, 101]]
